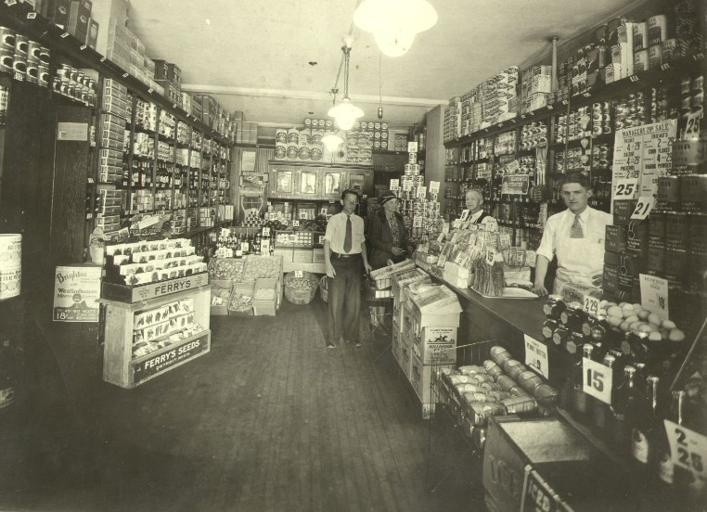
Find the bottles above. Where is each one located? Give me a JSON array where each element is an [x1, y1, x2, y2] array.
[[202, 173, 210, 189], [156, 162, 172, 188], [200, 190, 208, 207], [609, 365, 637, 442], [216, 231, 261, 257], [572, 344, 594, 414], [189, 170, 198, 189], [458, 182, 490, 200], [593, 353, 617, 433], [446, 184, 457, 199], [493, 184, 516, 202], [175, 166, 186, 188], [631, 375, 662, 470], [517, 194, 533, 223], [447, 200, 491, 217], [132, 160, 151, 186], [189, 190, 198, 207], [659, 391, 688, 486]]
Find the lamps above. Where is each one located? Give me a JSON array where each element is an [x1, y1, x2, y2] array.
[[353, 0, 439, 59], [320, 89, 342, 153], [327, 43, 366, 132]]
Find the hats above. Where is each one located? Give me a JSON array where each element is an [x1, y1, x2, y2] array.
[[378, 191, 396, 205]]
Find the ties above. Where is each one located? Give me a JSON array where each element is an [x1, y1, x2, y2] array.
[[343, 215, 352, 253], [570, 215, 584, 238]]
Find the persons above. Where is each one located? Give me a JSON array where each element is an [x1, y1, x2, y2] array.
[[460, 189, 498, 231], [531, 171, 612, 298], [321, 190, 372, 349], [368, 191, 412, 336]]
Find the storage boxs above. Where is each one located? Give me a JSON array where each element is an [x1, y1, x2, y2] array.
[[200, 250, 288, 319], [391, 267, 464, 409]]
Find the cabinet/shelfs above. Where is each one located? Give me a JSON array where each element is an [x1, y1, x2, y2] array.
[[267, 160, 376, 201], [268, 201, 365, 275]]
